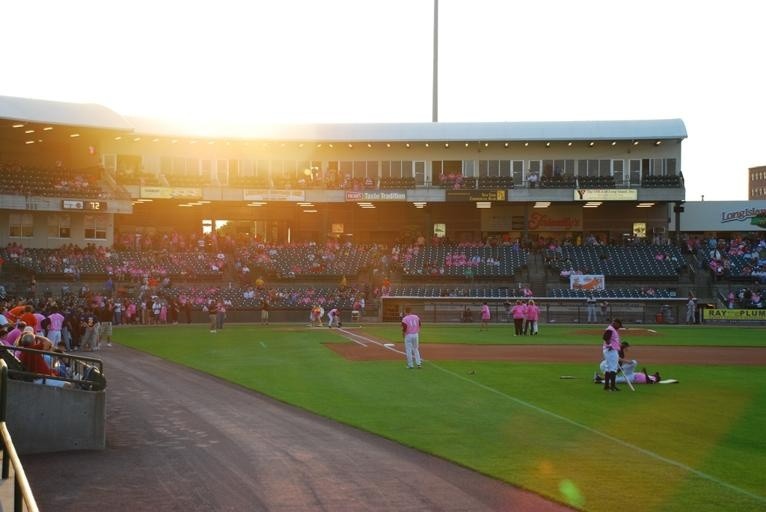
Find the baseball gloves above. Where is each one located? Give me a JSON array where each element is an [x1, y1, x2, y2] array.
[[338, 322, 343, 326]]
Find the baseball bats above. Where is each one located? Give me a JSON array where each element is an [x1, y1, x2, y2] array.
[[618, 363, 636, 391]]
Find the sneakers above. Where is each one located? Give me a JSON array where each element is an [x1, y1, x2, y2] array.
[[594, 370, 602, 383]]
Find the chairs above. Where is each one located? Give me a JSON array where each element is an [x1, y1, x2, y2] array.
[[1, 163, 684, 202], [3, 243, 766, 311]]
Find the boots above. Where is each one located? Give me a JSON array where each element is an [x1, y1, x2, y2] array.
[[604, 371, 612, 390], [610, 371, 621, 391]]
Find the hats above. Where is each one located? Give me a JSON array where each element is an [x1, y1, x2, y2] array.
[[613, 317, 624, 328], [656, 371, 661, 382]]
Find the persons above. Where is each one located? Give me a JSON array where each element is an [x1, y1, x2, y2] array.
[[601, 318, 624, 392], [259, 299, 270, 326], [593, 358, 661, 385], [479, 301, 491, 332], [310, 303, 323, 328], [616, 341, 631, 374], [326, 307, 342, 328], [519, 300, 528, 335], [206, 300, 217, 333], [401, 306, 424, 369], [521, 300, 538, 336], [532, 302, 541, 335], [0, 159, 766, 317], [0, 317, 114, 384], [509, 299, 528, 336], [216, 303, 226, 330]]
